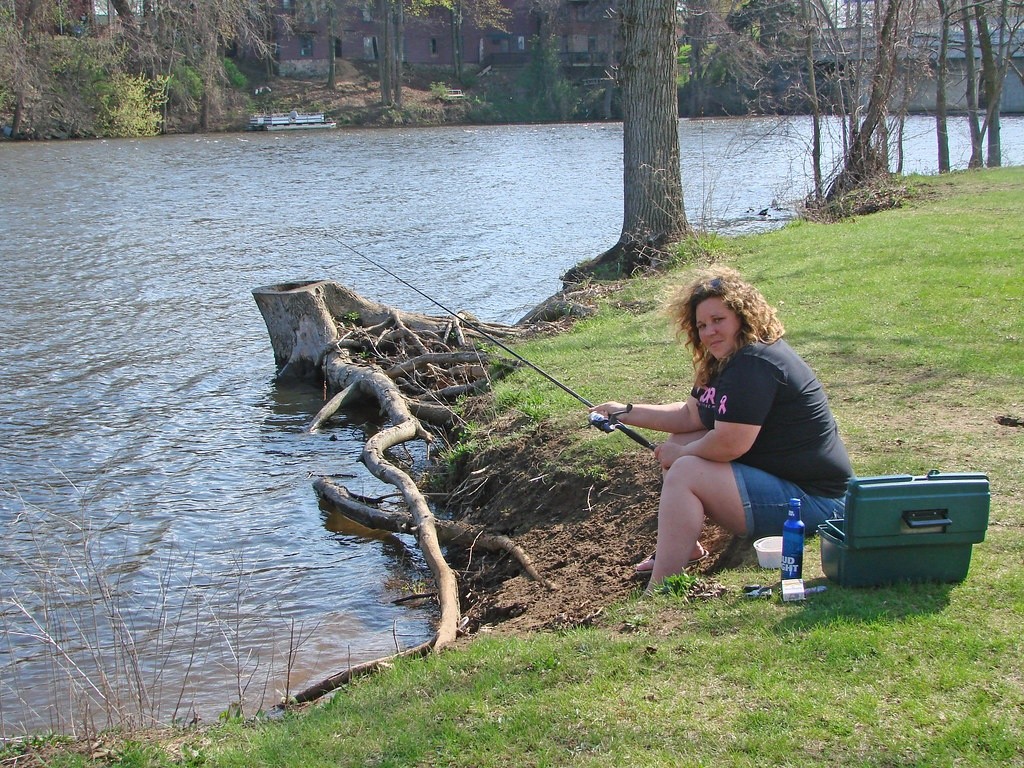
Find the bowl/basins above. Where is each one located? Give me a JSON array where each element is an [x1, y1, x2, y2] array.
[[753, 536, 789, 567]]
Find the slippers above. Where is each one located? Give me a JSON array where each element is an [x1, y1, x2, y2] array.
[[636, 549, 709, 573]]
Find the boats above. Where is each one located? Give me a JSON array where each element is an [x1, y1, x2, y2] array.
[[244, 112, 337, 132]]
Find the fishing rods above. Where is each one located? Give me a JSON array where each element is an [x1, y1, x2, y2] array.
[[323, 232, 655, 452]]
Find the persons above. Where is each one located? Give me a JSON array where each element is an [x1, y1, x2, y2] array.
[[588, 263, 854, 598]]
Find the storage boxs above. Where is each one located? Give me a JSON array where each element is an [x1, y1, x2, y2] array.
[[818, 469, 991, 586]]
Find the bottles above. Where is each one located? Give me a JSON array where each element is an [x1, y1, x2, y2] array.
[[780, 498, 805, 582]]
